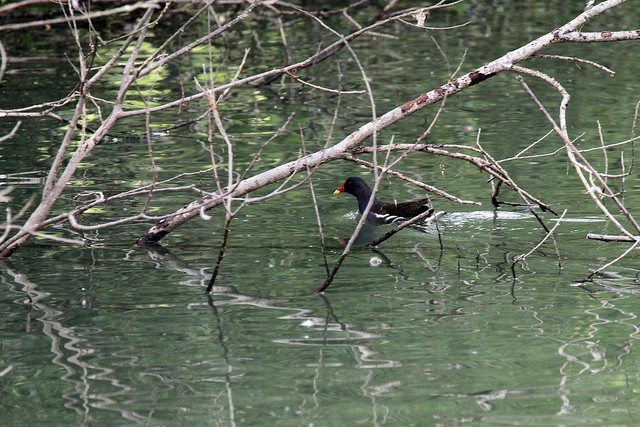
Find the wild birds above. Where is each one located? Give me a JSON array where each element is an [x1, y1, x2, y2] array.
[[331, 175, 448, 237]]
